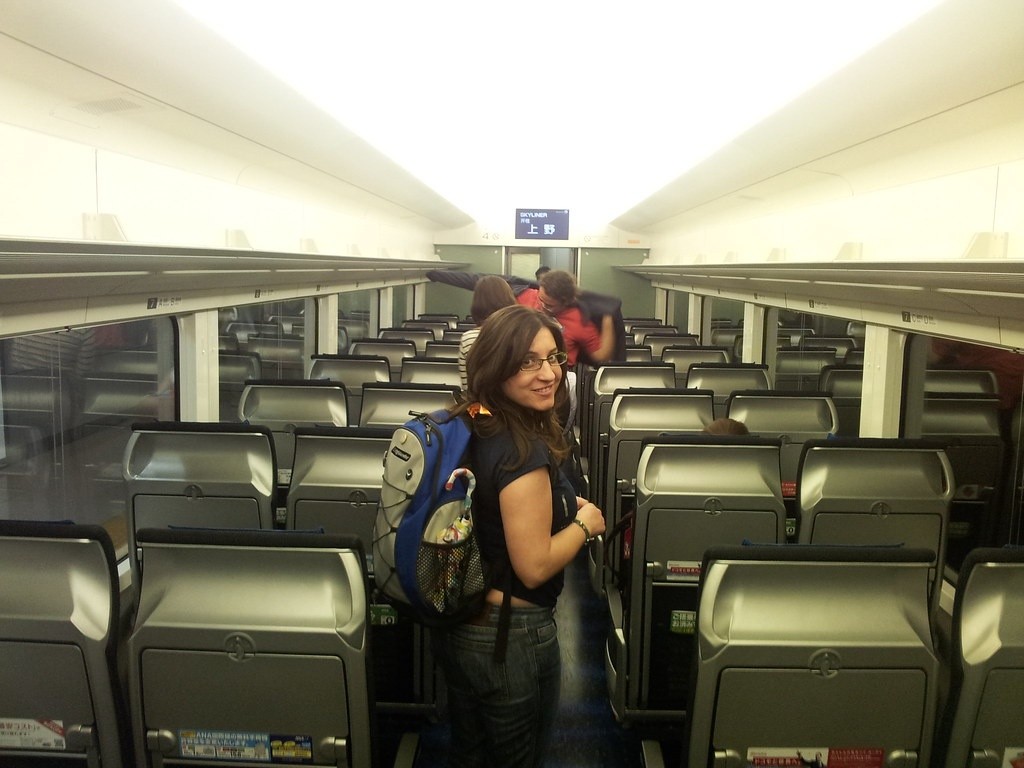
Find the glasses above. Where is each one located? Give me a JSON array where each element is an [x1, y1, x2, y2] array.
[[533, 296, 563, 313], [520, 351, 568, 371]]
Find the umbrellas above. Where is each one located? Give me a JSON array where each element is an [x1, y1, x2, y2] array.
[[431, 467, 477, 611]]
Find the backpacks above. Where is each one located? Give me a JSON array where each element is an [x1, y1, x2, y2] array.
[[370, 398, 494, 626]]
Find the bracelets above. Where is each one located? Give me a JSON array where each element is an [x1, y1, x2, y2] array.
[[571, 518, 591, 546]]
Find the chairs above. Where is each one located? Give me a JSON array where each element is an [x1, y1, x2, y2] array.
[[0, 307, 486, 768], [0, 296, 372, 524], [574, 317, 1024, 768], [709, 305, 1024, 586]]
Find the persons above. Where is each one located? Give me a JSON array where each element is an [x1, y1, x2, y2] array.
[[702, 419, 750, 438], [9, 322, 124, 388], [428, 304, 606, 768], [427, 266, 626, 504]]
